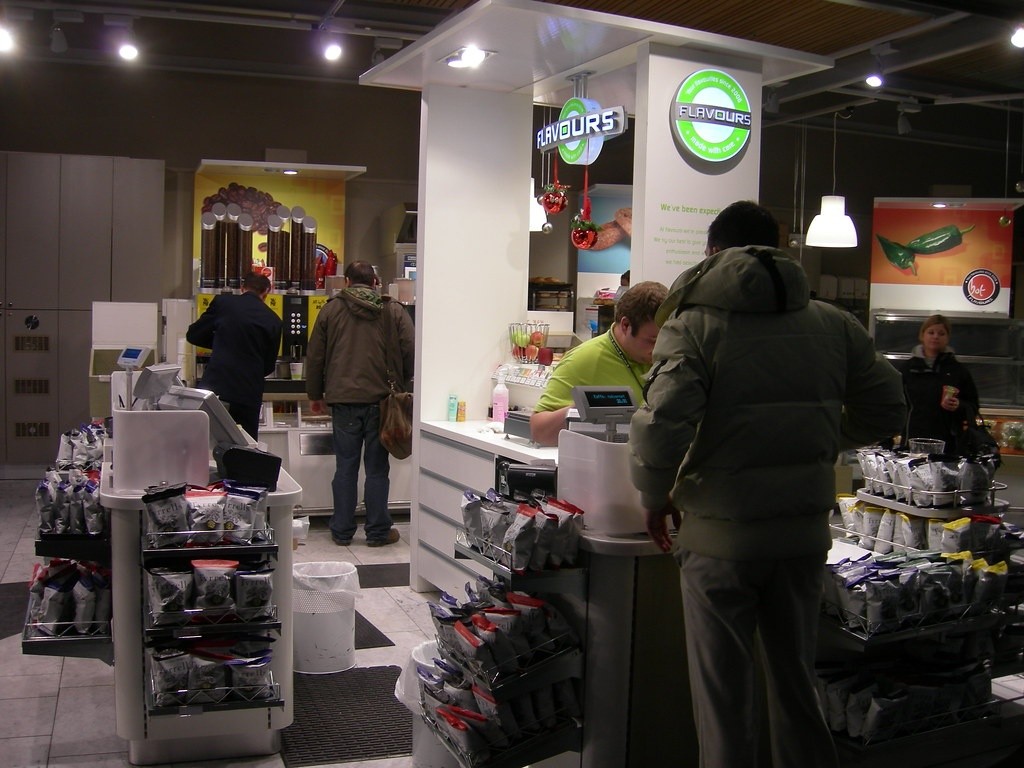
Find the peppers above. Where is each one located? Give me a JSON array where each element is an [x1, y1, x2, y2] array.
[[875, 233, 917, 276], [904, 225, 975, 254]]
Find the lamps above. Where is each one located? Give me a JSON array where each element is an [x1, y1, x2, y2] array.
[[805, 111, 859, 250], [896, 114, 913, 135], [765, 92, 780, 114]]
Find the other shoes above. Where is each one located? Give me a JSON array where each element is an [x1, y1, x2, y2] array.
[[329, 520, 353, 546], [367, 529, 399, 546]]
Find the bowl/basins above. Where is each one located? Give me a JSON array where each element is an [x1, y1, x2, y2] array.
[[507, 322, 550, 364]]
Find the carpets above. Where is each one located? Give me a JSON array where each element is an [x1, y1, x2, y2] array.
[[355, 562, 410, 589], [354, 609, 396, 650], [279, 664, 413, 768]]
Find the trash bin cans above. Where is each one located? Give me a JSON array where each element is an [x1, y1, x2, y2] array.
[[294, 559, 358, 674]]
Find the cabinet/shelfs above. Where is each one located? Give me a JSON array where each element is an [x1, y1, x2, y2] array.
[[418, 524, 592, 768], [21, 530, 115, 668], [139, 520, 286, 720], [820, 478, 1016, 753], [1, 151, 166, 481]]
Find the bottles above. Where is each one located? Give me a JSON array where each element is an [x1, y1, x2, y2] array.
[[449, 393, 466, 422], [389, 284, 398, 301], [492, 376, 509, 432]]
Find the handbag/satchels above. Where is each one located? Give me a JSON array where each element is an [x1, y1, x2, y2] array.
[[967, 411, 1002, 470], [378, 391, 413, 459]]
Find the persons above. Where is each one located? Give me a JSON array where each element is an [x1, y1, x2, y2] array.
[[187, 274, 283, 445], [896, 315, 979, 460], [529, 281, 676, 446], [629, 201, 906, 768], [304, 260, 414, 545]]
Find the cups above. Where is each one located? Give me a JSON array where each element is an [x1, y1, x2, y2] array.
[[290, 363, 303, 380], [538, 347, 553, 366], [941, 385, 959, 406], [908, 439, 945, 454]]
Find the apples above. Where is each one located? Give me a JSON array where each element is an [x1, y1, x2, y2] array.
[[511, 331, 553, 366]]
